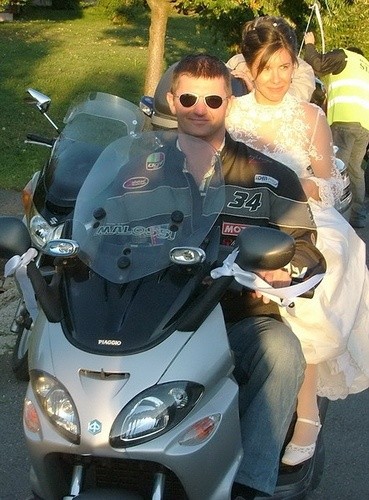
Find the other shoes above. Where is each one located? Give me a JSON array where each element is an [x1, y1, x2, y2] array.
[[349, 211, 366, 229]]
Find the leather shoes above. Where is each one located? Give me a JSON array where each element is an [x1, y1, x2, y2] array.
[[282, 418, 322, 466]]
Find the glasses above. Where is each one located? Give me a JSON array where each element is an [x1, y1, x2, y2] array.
[[170, 90, 229, 110]]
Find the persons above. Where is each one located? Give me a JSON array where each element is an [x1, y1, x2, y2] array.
[[225, 19, 343, 466], [60, 53, 327, 500], [304, 32, 369, 228], [225, 53, 316, 102]]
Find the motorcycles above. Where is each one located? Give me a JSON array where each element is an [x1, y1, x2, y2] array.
[[0, 128, 353, 500], [8, 59, 251, 382]]
